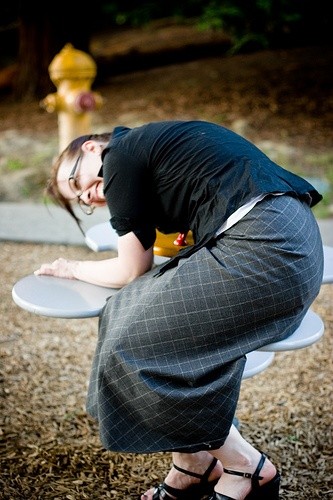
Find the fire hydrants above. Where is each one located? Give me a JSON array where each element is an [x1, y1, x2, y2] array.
[[38, 42, 107, 154]]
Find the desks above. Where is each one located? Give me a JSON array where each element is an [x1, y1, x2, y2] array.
[[11, 222, 333, 425]]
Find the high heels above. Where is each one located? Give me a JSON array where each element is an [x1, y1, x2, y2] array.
[[202, 454, 280, 500], [133, 451, 222, 500]]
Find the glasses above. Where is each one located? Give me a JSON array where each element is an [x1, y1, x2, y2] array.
[[68, 134, 96, 215]]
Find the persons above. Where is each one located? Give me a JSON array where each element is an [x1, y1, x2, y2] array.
[[43, 120, 324, 500]]
